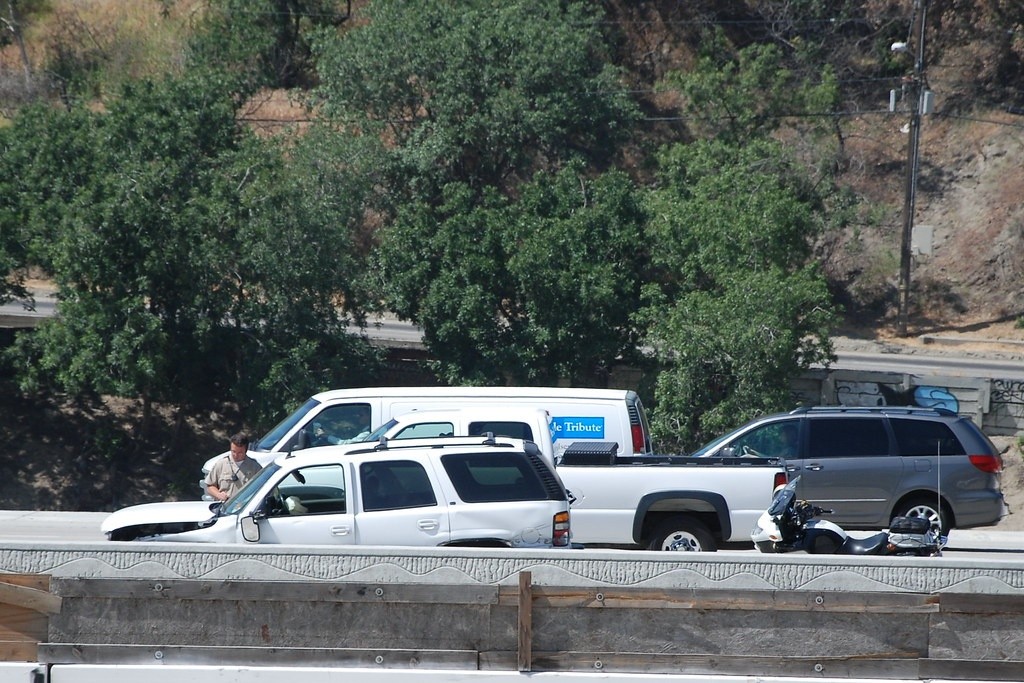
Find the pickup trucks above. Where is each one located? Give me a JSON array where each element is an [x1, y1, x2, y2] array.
[[358, 407, 792, 555]]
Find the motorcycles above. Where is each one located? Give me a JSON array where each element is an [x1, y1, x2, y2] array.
[[754, 475, 946, 557]]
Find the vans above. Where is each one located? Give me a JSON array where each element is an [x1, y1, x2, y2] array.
[[200, 387, 651, 507]]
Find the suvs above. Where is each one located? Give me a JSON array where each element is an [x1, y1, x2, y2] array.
[[99, 431, 574, 556], [681, 405, 1010, 542]]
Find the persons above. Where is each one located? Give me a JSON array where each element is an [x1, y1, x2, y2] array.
[[204, 432, 263, 502], [317, 406, 372, 445]]
